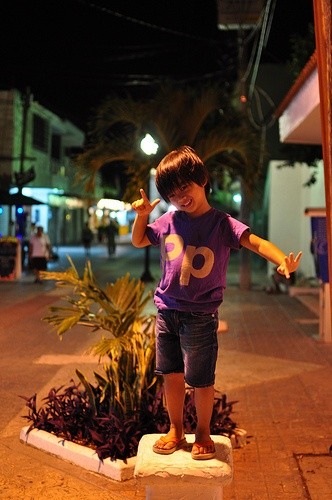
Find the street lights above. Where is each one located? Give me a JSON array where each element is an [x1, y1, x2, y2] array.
[[139, 131, 157, 282]]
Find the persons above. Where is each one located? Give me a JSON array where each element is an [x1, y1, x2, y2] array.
[[131, 144, 303, 461], [81, 221, 94, 256], [104, 220, 120, 260], [29, 226, 53, 284]]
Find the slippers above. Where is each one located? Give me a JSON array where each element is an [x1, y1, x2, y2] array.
[[191, 440, 216, 460], [152, 436, 186, 454]]
